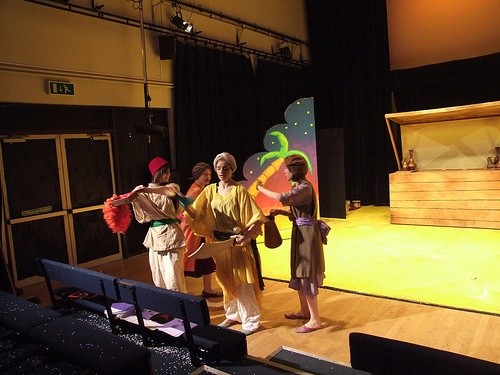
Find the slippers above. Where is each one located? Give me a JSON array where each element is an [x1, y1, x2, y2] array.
[[296, 324, 321, 333], [285, 311, 311, 320]]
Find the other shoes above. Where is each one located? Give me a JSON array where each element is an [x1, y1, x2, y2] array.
[[201, 289, 224, 299], [240, 328, 255, 335], [217, 318, 239, 328]]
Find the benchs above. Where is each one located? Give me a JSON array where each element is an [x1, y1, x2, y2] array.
[[36, 257, 247, 367], [349, 332, 500, 375]]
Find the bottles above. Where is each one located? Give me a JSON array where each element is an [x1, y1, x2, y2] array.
[[402, 157, 408, 170], [345, 200, 350, 214], [494, 147, 500, 165], [408, 150, 416, 170]]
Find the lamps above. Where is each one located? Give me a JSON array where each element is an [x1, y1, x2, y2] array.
[[170, 12, 192, 33]]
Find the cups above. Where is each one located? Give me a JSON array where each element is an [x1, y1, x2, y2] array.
[[352, 201, 361, 208]]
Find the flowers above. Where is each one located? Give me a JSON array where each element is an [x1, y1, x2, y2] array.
[[104, 196, 133, 234]]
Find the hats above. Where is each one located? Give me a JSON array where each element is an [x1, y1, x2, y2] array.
[[192, 163, 211, 181], [213, 152, 238, 173], [148, 157, 170, 176], [284, 154, 308, 182]]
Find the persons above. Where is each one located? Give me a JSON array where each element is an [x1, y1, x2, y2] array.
[[178, 162, 224, 298], [257, 154, 330, 333], [111, 157, 186, 295], [172, 152, 270, 335]]
